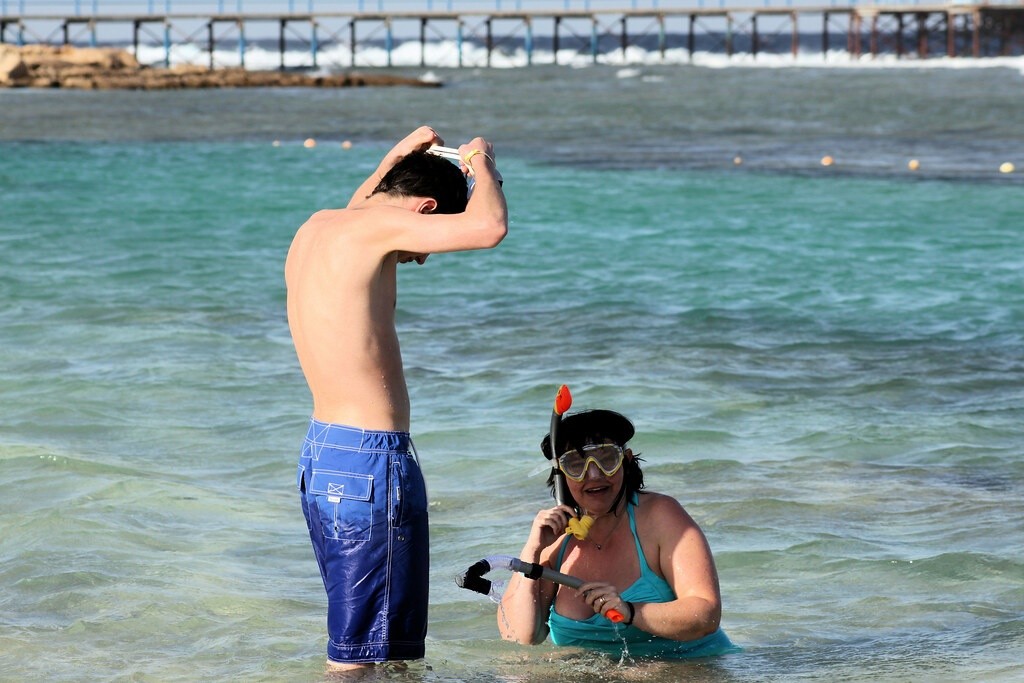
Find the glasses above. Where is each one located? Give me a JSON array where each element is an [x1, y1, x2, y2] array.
[[426, 145, 504, 196], [559, 444, 623, 482]]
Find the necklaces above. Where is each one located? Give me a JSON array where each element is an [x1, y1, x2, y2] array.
[[586, 498, 625, 550]]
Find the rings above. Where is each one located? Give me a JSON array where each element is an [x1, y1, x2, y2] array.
[[598, 595, 608, 604]]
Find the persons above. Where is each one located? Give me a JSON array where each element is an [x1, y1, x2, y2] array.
[[280, 125, 509, 671], [495, 409, 745, 661]]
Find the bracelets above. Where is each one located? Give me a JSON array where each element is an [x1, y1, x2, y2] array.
[[463, 147, 496, 172]]
